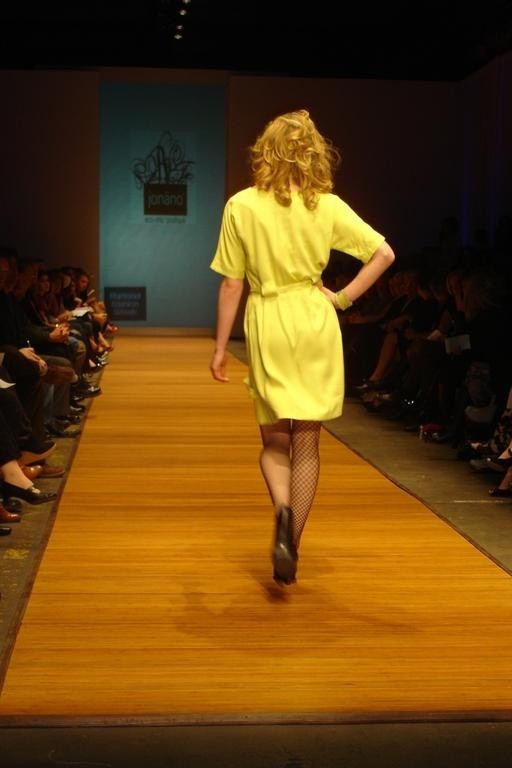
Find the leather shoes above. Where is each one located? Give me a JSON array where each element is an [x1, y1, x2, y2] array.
[[46, 350, 109, 433], [472, 441, 512, 497], [0, 438, 65, 535]]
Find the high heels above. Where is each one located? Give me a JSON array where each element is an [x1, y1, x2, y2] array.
[[273, 508, 298, 585]]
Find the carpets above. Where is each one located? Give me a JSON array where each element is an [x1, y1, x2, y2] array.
[[0, 334, 512, 731]]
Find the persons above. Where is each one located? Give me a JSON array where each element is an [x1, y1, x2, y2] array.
[[0, 248, 117, 536], [208, 108, 395, 585], [330, 252, 511, 496]]
